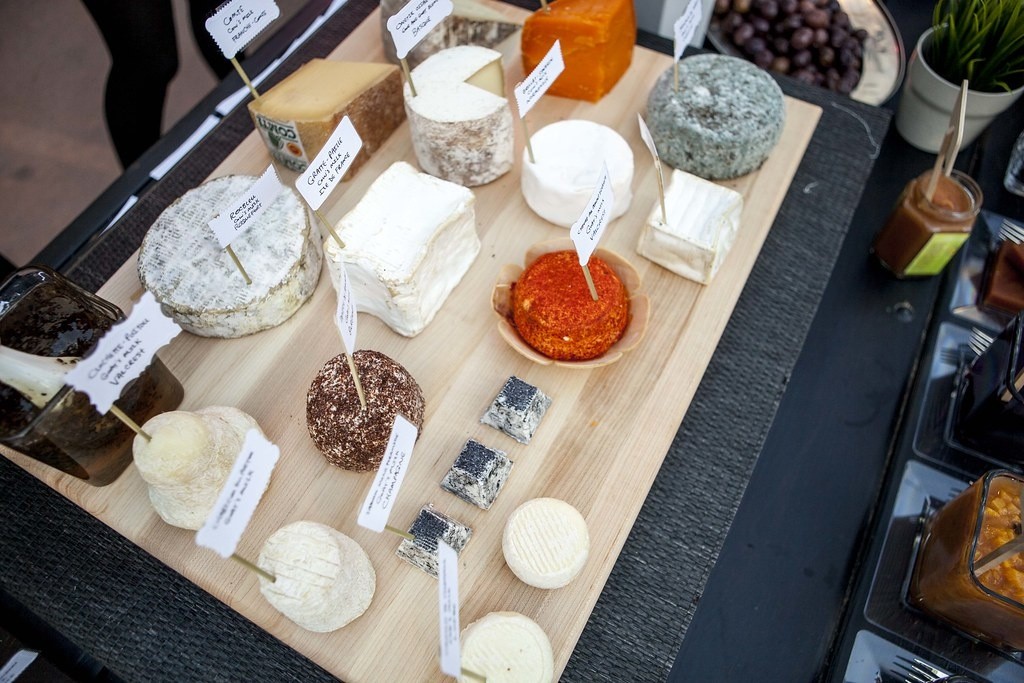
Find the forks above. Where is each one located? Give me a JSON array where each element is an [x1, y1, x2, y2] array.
[[966, 326, 993, 356], [997, 218, 1024, 250], [890, 654, 948, 683]]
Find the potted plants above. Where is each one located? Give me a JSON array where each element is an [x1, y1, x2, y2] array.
[[883, 0, 1024, 153]]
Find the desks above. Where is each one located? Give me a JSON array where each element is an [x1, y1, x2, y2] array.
[[0, 0, 1024, 682]]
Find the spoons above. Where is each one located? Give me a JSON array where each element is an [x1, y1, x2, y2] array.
[[0, 344, 84, 413]]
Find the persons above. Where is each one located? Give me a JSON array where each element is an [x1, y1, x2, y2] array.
[[80, 0, 248, 172]]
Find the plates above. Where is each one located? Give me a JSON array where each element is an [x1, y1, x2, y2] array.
[[705, 0, 906, 105]]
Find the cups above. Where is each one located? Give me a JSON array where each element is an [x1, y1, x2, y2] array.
[[942, 676, 976, 683], [950, 310, 1024, 468], [905, 467, 1024, 653], [0, 264, 186, 487]]
